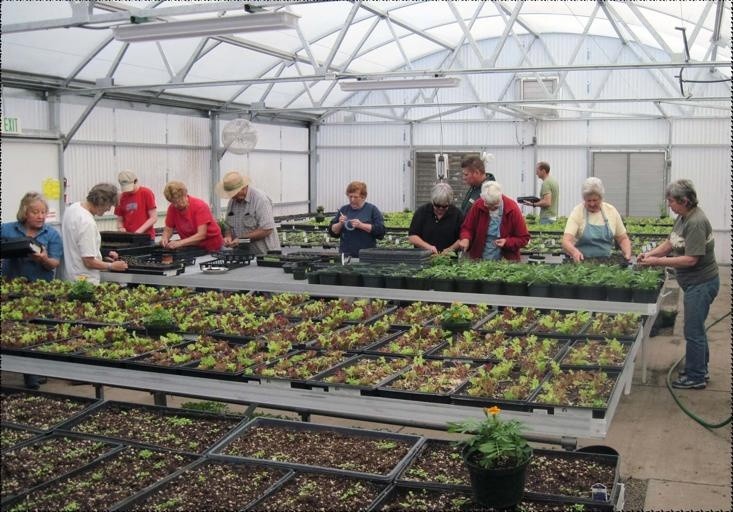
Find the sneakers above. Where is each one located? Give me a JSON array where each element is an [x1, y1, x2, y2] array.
[[672, 370, 709, 389]]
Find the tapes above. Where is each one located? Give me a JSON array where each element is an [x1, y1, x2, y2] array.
[[344, 220, 355, 231]]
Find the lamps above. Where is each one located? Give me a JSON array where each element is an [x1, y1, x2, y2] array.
[[112, 12, 300, 43], [338, 76, 462, 92]]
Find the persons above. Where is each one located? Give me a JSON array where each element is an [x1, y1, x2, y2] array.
[[523, 160, 559, 224], [0, 190, 64, 283], [459, 156, 496, 216], [559, 176, 632, 264], [406, 182, 464, 255], [456, 179, 532, 263], [113, 168, 158, 244], [56, 183, 130, 283], [327, 180, 386, 257], [213, 170, 282, 257], [635, 177, 721, 389], [159, 178, 225, 254]]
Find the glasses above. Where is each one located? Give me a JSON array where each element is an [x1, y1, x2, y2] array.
[[433, 200, 448, 208]]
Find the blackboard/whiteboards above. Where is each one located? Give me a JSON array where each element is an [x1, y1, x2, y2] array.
[[0, 139, 63, 227]]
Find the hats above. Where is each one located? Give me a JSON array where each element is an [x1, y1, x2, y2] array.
[[215, 171, 252, 199], [118, 170, 137, 193]]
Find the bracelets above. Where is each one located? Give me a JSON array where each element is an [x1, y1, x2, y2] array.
[[108, 262, 113, 271], [532, 202, 534, 208]]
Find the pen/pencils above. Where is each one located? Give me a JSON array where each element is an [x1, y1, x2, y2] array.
[[338, 209, 343, 216]]
